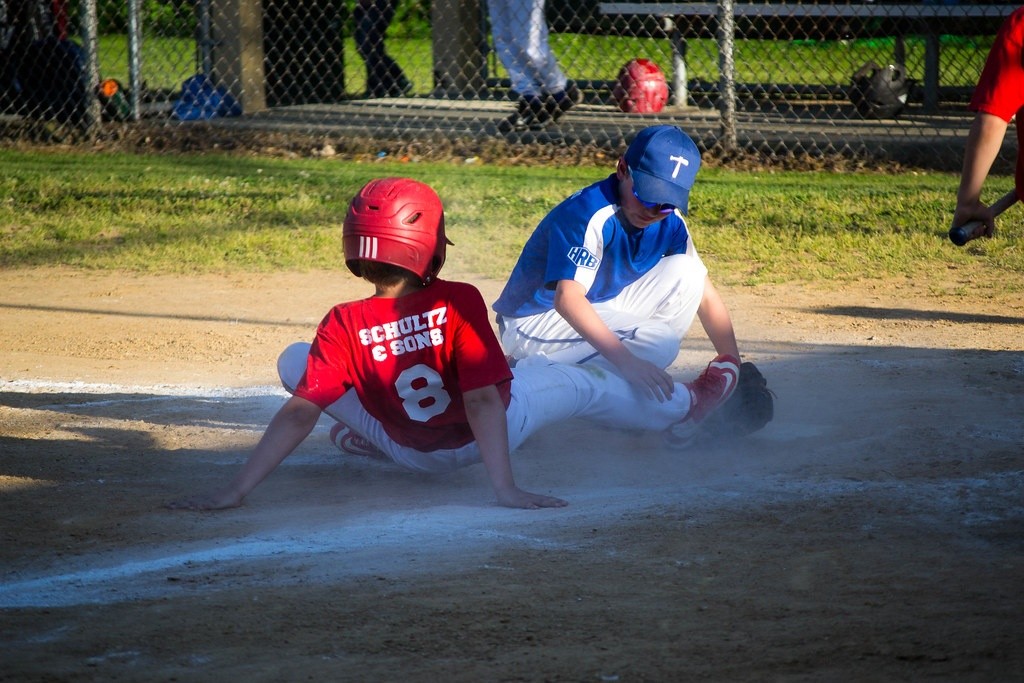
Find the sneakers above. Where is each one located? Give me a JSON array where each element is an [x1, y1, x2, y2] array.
[[499, 80, 583, 132], [330, 422, 396, 465], [663, 354, 739, 449]]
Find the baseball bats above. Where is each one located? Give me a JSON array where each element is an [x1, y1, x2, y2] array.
[[949, 188, 1022, 247]]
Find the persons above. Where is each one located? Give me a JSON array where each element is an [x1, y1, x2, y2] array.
[[166, 177, 737, 510], [493, 125, 741, 440], [353, 0, 413, 98], [487, 0, 583, 134], [952, 4, 1024, 239]]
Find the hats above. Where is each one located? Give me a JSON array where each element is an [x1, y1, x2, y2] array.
[[625, 125, 701, 217]]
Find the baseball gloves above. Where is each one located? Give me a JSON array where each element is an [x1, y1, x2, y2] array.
[[704, 361, 775, 437]]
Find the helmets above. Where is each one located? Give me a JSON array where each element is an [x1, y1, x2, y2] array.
[[849, 62, 907, 120], [613, 58, 669, 115], [342, 178, 455, 287]]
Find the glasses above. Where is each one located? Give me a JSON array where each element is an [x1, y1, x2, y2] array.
[[628, 166, 676, 215]]
